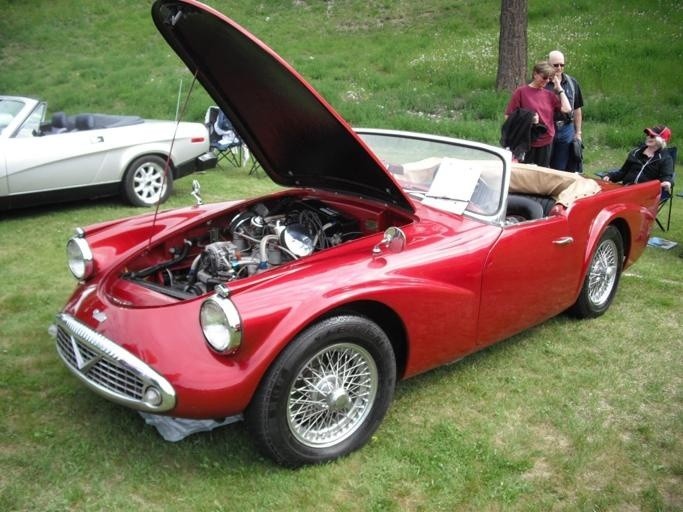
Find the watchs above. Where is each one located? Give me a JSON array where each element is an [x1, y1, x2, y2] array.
[[575, 130, 581, 134]]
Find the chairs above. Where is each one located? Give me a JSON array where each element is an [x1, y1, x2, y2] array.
[[594, 146, 677, 233], [210, 108, 242, 168], [249, 151, 269, 177]]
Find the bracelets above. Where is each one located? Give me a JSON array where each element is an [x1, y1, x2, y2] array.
[[555, 87, 564, 94]]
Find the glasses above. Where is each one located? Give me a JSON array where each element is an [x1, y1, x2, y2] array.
[[552, 63, 564, 67], [537, 73, 553, 81], [645, 133, 655, 139]]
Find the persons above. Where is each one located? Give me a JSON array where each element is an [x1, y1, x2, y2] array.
[[545, 50, 583, 174], [504, 60, 571, 169], [601, 125, 674, 203]]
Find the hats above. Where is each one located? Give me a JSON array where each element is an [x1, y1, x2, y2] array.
[[643, 124, 671, 146]]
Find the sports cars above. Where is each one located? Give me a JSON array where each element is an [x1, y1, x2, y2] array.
[[0, 96, 208, 208], [54, 1, 661, 468]]
